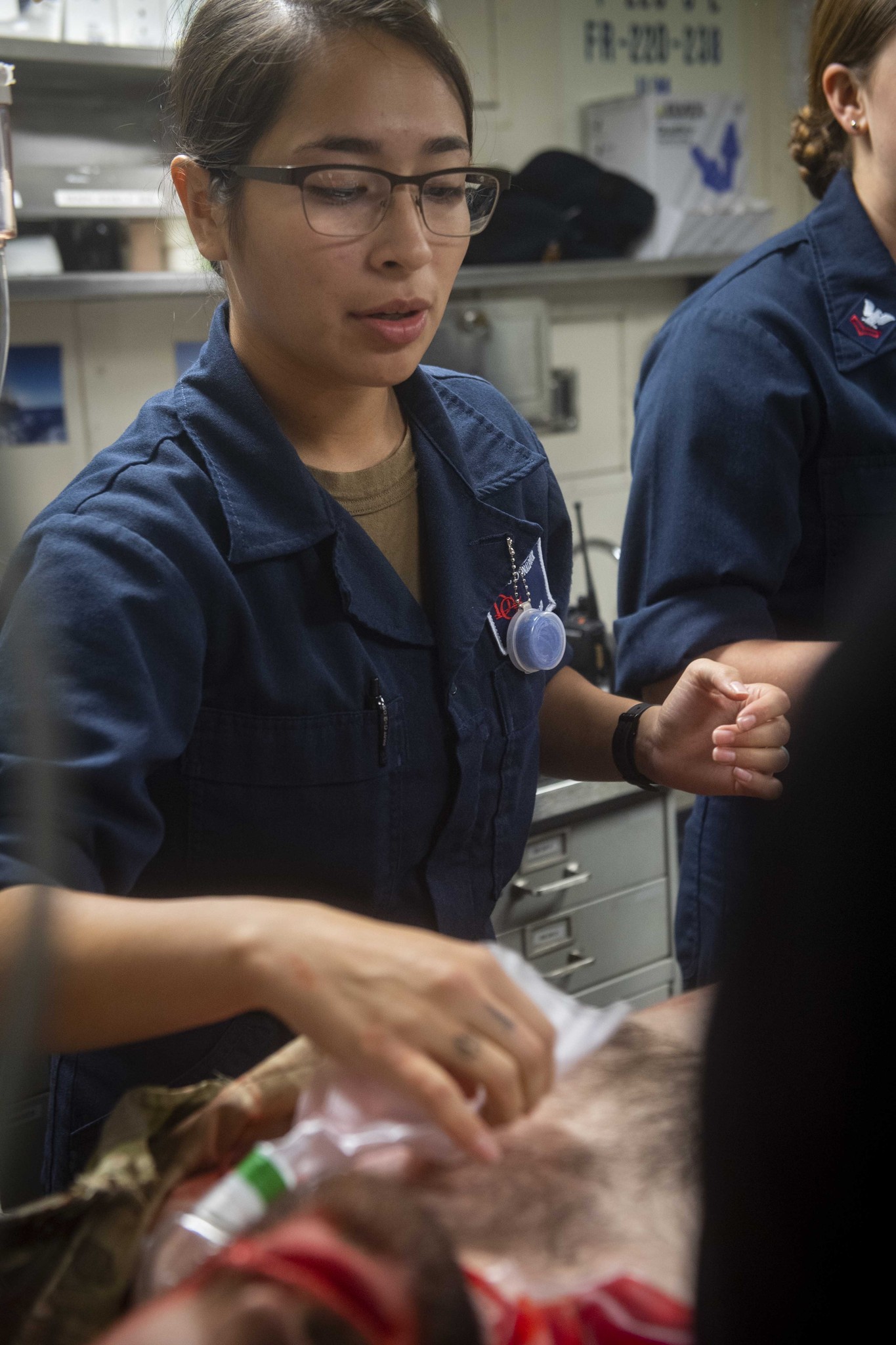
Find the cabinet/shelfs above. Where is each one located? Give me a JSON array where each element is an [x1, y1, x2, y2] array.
[[473, 773, 697, 1023]]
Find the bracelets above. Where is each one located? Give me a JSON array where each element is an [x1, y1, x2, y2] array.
[[611, 701, 664, 792]]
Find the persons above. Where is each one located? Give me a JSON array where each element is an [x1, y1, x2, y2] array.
[[0, 0, 792, 1164], [601, 0, 895, 1344]]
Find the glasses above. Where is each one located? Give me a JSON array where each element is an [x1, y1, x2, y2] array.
[[195, 159, 509, 239]]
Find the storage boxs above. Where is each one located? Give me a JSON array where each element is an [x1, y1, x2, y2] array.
[[570, 86, 756, 269]]
